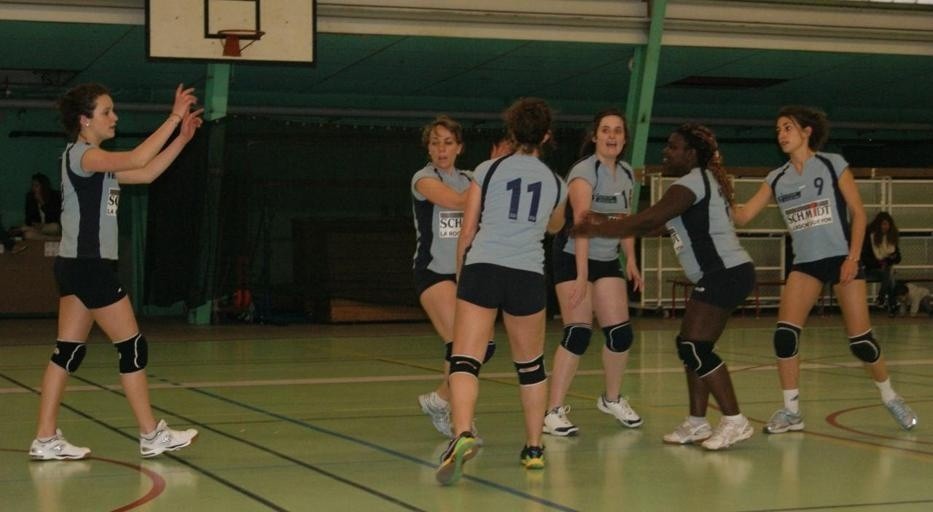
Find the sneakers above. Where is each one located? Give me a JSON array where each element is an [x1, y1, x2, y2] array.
[[28, 427, 92, 461], [137, 418, 199, 458]]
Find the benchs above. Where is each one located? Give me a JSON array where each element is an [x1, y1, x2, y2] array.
[[668, 276, 933, 320]]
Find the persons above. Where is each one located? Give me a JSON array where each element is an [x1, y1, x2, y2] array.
[[22, 172, 61, 241], [580, 122, 756, 452], [410, 114, 511, 441], [730, 106, 917, 436], [541, 108, 644, 437], [861, 212, 902, 317], [894, 279, 933, 319], [29, 81, 205, 462], [435, 97, 570, 487]]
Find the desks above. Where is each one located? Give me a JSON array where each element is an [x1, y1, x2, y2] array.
[[0, 234, 133, 318]]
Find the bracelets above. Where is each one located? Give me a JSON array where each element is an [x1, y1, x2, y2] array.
[[170, 112, 182, 121], [845, 255, 860, 263]]
